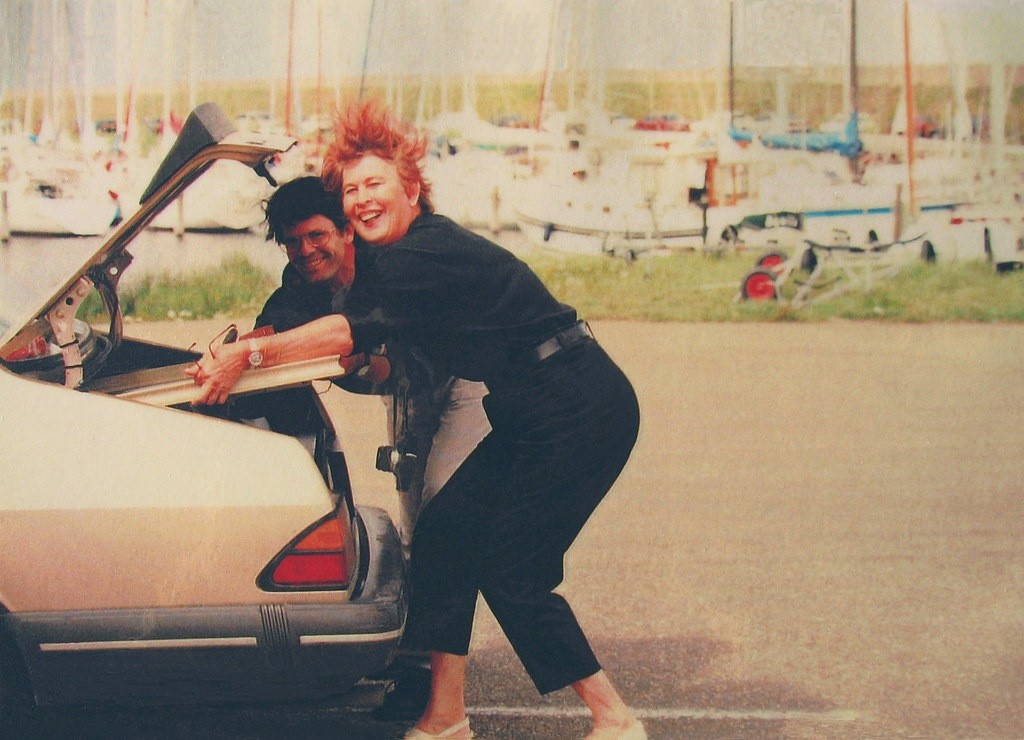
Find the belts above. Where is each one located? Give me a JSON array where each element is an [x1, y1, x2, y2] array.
[[515, 322, 590, 375]]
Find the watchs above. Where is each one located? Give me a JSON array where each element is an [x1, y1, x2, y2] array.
[[248, 338, 263, 370], [355, 352, 370, 376]]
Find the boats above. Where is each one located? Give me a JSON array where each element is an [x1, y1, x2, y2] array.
[[2, 2, 1024, 269]]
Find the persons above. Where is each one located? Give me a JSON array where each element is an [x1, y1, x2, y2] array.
[[186, 108, 650, 740], [253, 175, 491, 720]]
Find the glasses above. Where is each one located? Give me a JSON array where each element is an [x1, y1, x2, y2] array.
[[280, 227, 338, 253], [187, 323, 239, 369]]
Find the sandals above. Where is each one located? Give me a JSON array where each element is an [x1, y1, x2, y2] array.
[[583, 721, 648, 740], [403, 716, 473, 740]]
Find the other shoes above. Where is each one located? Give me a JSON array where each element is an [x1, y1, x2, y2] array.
[[366, 666, 431, 720], [360, 654, 423, 684]]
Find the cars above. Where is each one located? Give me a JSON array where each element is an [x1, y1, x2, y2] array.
[[0, 99, 425, 739]]
